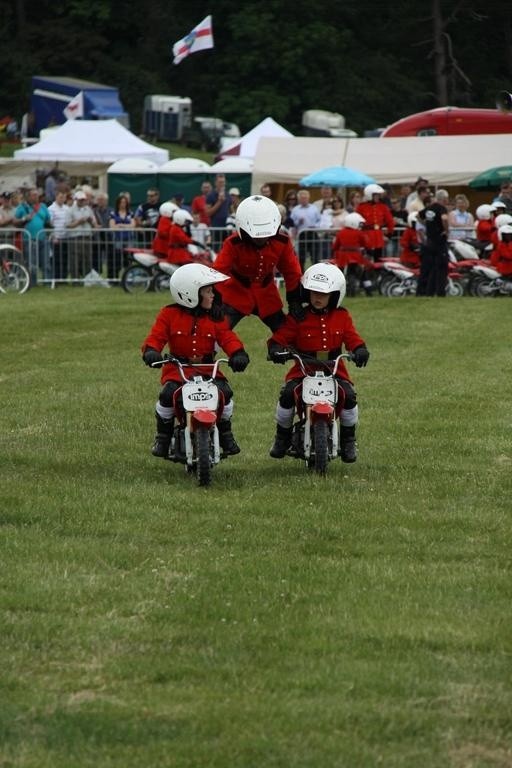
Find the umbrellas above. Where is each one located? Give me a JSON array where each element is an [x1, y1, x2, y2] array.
[[299, 166, 377, 209], [468, 166, 512, 192]]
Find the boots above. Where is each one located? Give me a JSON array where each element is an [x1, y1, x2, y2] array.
[[218, 422, 240, 454], [151, 416, 174, 457], [269, 425, 294, 458], [340, 425, 356, 462]]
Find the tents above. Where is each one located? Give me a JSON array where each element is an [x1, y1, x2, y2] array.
[[214, 117, 294, 163], [251, 133, 512, 197], [14, 117, 169, 162]]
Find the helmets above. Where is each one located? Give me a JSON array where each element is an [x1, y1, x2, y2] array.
[[363, 184, 385, 203], [299, 262, 346, 309], [344, 212, 366, 229], [235, 195, 282, 241], [159, 201, 179, 218], [497, 225, 512, 242], [173, 210, 194, 227], [475, 204, 497, 221], [407, 211, 426, 230], [494, 213, 512, 230], [491, 201, 506, 212], [170, 263, 231, 310]]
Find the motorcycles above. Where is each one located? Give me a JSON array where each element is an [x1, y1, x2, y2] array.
[[342, 247, 366, 296], [0, 244, 30, 296], [374, 257, 401, 294], [469, 264, 512, 297], [268, 352, 356, 476], [152, 356, 230, 487], [123, 247, 156, 296], [385, 246, 463, 298], [156, 246, 219, 294]]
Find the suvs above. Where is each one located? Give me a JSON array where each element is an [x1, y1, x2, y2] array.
[[181, 116, 240, 152]]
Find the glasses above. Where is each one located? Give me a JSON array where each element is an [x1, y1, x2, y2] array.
[[145, 192, 158, 198], [287, 197, 298, 201]]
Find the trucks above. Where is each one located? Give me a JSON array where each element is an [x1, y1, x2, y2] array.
[[29, 75, 129, 137]]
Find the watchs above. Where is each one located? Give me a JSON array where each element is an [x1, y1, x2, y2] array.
[[22, 216, 26, 222]]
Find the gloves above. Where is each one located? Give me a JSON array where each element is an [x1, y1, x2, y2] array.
[[142, 346, 163, 368], [268, 342, 286, 365], [228, 349, 250, 372], [352, 345, 369, 367], [288, 297, 305, 321], [209, 299, 224, 321]]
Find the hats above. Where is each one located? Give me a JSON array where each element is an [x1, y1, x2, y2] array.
[[413, 175, 429, 186], [229, 187, 239, 195], [72, 191, 87, 200]]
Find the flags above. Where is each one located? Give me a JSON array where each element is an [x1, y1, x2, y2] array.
[[64, 92, 83, 120], [172, 14, 214, 65]]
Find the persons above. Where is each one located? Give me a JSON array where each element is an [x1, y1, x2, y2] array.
[[74, 173, 89, 190], [134, 187, 163, 248], [277, 204, 296, 247], [332, 212, 375, 298], [406, 186, 430, 213], [491, 201, 506, 218], [491, 214, 512, 249], [493, 184, 512, 217], [13, 190, 54, 287], [68, 173, 78, 191], [0, 191, 17, 264], [490, 225, 512, 276], [192, 181, 213, 227], [204, 173, 232, 253], [64, 191, 97, 287], [228, 188, 245, 214], [207, 195, 306, 361], [290, 190, 321, 275], [448, 195, 474, 239], [261, 185, 279, 206], [319, 200, 333, 214], [399, 183, 412, 209], [46, 168, 67, 205], [108, 196, 136, 286], [357, 184, 395, 263], [92, 192, 112, 274], [345, 191, 361, 213], [172, 192, 192, 214], [283, 190, 299, 217], [312, 187, 335, 213], [416, 190, 448, 297], [168, 209, 209, 266], [386, 194, 409, 257], [48, 190, 70, 285], [379, 183, 395, 208], [400, 211, 420, 269], [154, 201, 180, 258], [267, 263, 369, 463], [416, 196, 435, 297], [405, 176, 428, 207], [141, 263, 249, 456], [310, 195, 348, 265], [476, 204, 495, 241]]
